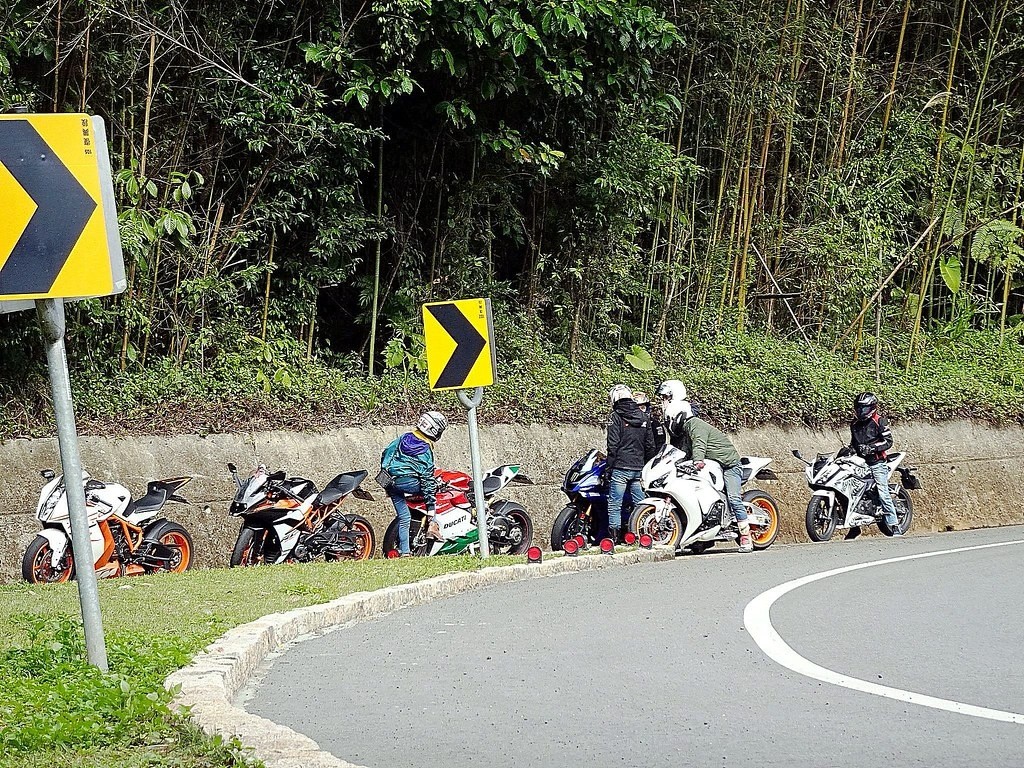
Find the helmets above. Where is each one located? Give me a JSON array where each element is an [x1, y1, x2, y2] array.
[[416, 410, 447, 439], [664, 401, 694, 436], [657, 380, 687, 402], [610, 384, 632, 408], [854, 392, 877, 422], [632, 392, 649, 405]]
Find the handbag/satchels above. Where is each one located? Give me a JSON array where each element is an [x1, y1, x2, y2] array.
[[376, 471, 394, 490]]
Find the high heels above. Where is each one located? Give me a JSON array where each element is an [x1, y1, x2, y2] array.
[[426, 524, 444, 542]]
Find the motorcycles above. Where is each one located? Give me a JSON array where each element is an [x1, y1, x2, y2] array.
[[383, 462, 535, 559], [551, 449, 635, 552], [22, 469, 194, 584], [628, 443, 781, 550], [228, 463, 376, 568], [792, 446, 922, 542]]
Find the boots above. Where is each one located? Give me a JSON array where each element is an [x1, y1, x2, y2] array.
[[738, 519, 753, 552]]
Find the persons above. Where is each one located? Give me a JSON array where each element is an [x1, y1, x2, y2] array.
[[604, 384, 657, 545], [380, 411, 448, 557], [632, 391, 666, 454], [842, 392, 903, 540], [658, 379, 703, 450], [665, 399, 754, 553]]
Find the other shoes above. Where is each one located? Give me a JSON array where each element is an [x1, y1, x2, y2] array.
[[845, 526, 861, 540], [892, 525, 901, 536]]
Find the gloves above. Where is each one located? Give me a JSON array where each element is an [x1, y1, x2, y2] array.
[[693, 461, 704, 470], [603, 469, 610, 487], [867, 443, 878, 457]]
